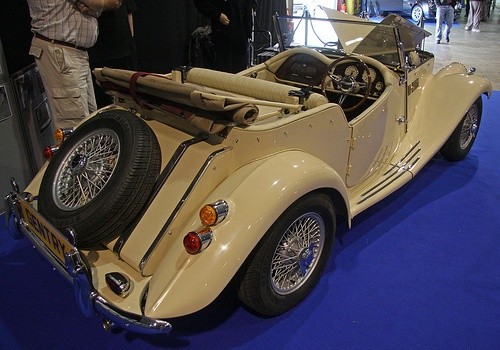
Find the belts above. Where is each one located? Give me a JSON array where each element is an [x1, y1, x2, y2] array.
[[35, 33, 87, 51]]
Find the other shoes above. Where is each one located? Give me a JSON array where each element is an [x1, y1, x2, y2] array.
[[465, 27, 470, 30], [445, 37, 449, 43], [435, 40, 440, 44], [471, 28, 480, 32]]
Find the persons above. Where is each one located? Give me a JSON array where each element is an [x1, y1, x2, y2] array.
[[435, 0, 456, 44], [193, 0, 258, 74], [465, 0, 485, 32], [26, 0, 137, 131]]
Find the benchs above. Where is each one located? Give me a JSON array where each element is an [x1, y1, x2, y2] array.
[[186, 68, 330, 111]]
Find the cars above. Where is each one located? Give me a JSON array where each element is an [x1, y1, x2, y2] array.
[[375, 0, 462, 23], [2, 4, 493, 336]]
